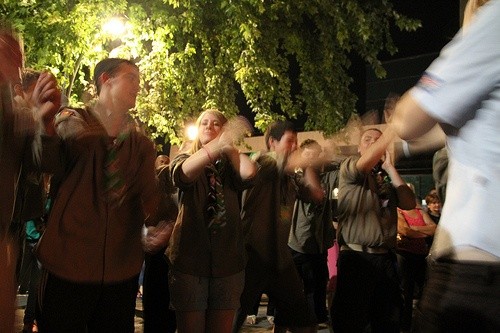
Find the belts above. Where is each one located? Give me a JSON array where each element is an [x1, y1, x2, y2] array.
[[428, 259, 500, 284], [340, 243, 396, 254]]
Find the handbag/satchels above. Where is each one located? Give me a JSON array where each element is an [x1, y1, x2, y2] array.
[[396, 235, 429, 255]]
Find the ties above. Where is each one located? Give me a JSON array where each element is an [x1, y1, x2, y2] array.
[[104, 129, 128, 207], [205, 160, 226, 234]]
